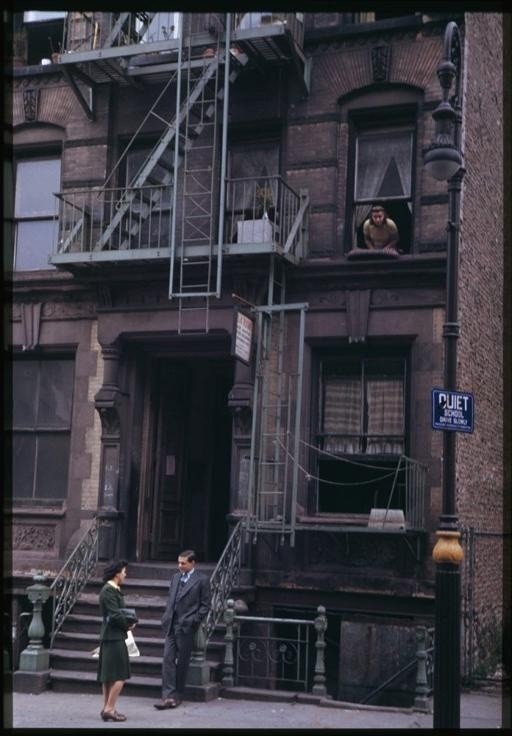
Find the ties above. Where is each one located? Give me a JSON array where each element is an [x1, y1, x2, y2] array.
[[180, 574, 188, 582]]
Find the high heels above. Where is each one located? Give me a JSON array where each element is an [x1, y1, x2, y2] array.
[[100, 708, 127, 722]]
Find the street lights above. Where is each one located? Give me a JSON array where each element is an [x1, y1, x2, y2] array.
[[416, 19, 465, 729]]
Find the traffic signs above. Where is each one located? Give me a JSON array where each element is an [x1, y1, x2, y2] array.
[[432, 389, 474, 433]]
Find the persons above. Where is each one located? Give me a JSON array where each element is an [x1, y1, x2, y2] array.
[[152, 548, 212, 710], [361, 205, 400, 252], [94, 557, 138, 723]]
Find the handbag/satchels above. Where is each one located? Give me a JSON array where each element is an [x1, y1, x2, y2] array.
[[106, 608, 138, 627]]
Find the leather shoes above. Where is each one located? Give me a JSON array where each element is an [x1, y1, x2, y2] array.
[[153, 697, 182, 709]]
